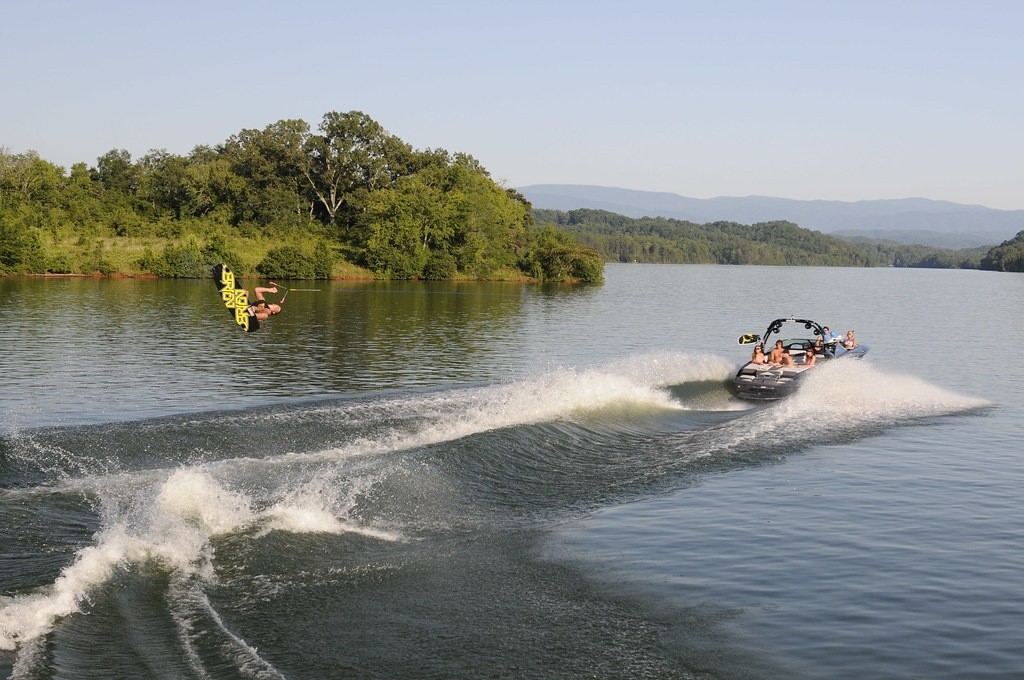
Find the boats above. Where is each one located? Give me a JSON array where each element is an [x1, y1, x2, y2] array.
[[732, 314, 871, 401]]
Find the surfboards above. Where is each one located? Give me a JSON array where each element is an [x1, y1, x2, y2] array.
[[212, 262, 260, 333]]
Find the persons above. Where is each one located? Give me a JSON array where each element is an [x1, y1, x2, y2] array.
[[752, 339, 816, 369], [241, 286, 283, 324], [815, 327, 857, 351]]
